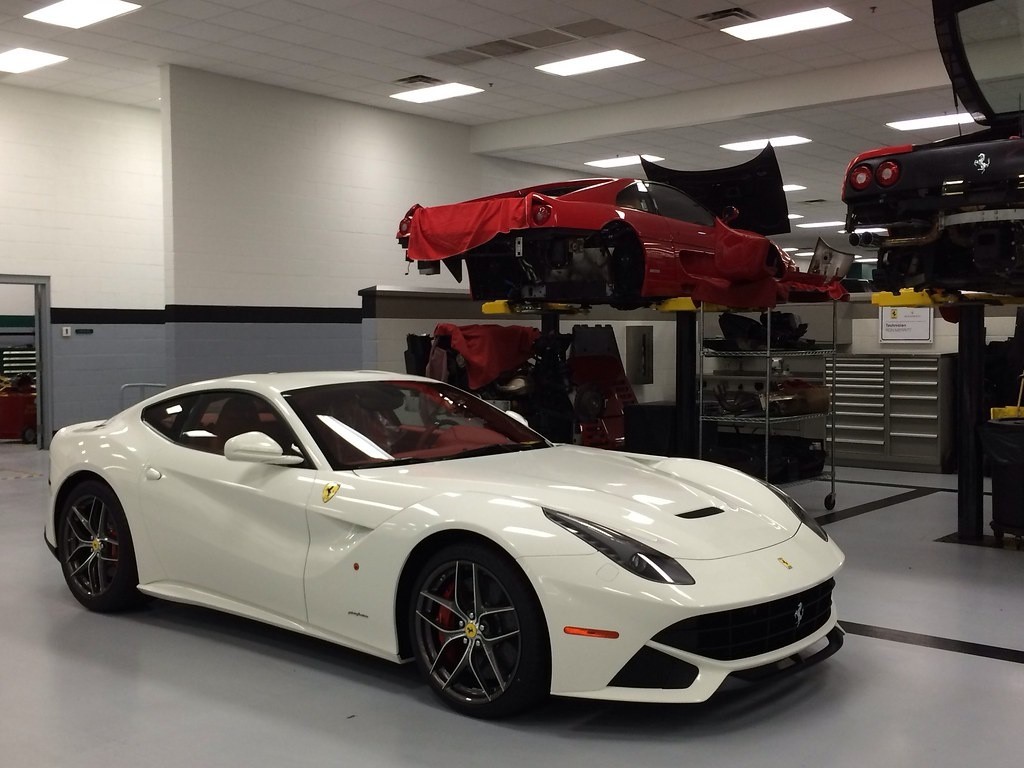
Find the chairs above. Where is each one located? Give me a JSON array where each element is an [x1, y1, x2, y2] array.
[[311, 397, 390, 458], [212, 399, 263, 457]]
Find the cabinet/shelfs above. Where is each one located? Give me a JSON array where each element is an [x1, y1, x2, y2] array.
[[695, 301, 837, 509]]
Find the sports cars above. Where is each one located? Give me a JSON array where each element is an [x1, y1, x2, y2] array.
[[837, 0, 1024, 296], [38, 369, 846, 722], [394, 140, 792, 313]]
